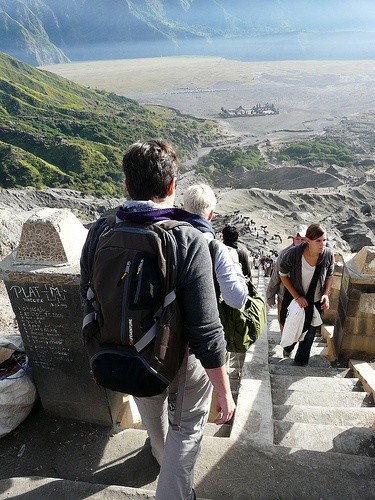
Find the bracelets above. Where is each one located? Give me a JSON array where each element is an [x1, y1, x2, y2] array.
[[295, 295, 301, 300]]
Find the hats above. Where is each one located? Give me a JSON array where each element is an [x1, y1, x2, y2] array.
[[288, 224, 308, 239]]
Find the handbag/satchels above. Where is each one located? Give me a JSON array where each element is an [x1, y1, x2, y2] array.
[[218, 276, 266, 353]]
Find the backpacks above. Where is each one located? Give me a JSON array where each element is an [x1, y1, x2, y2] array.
[[81, 206, 194, 398]]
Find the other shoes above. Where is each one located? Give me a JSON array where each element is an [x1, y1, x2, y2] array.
[[144, 437, 161, 470], [192, 488, 196, 500], [283, 350, 291, 357]]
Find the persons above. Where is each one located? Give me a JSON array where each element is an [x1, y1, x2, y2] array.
[[165, 182, 249, 426], [78, 136, 237, 500], [220, 225, 251, 281], [278, 223, 335, 367], [265, 224, 309, 333], [251, 249, 280, 280]]
